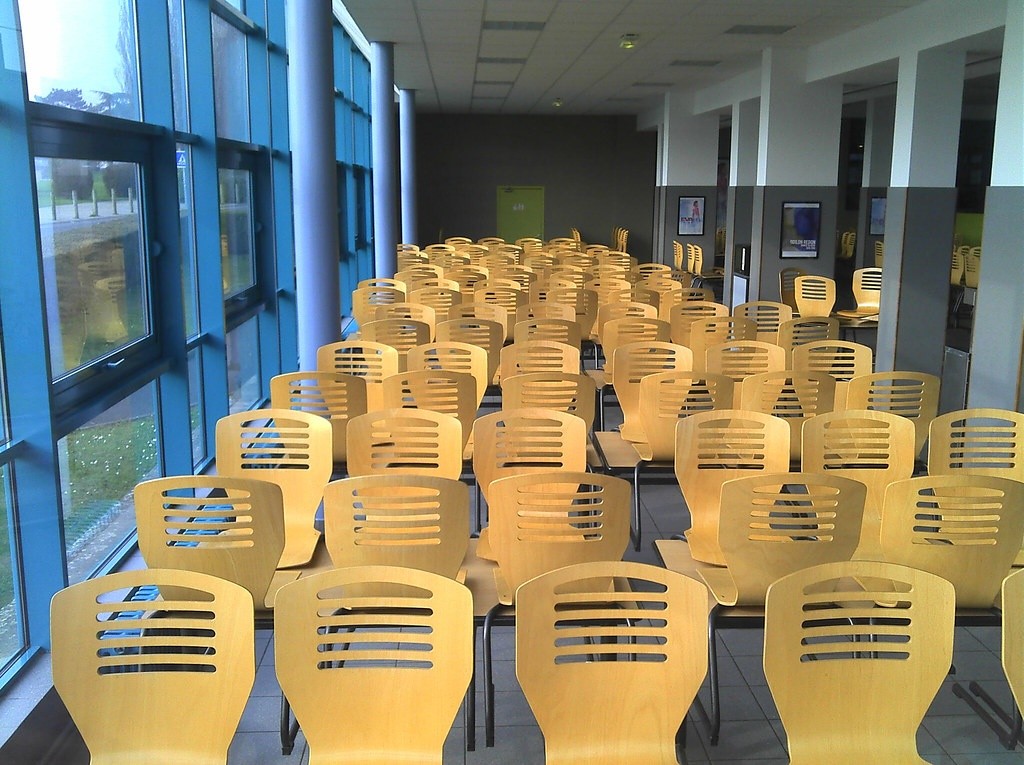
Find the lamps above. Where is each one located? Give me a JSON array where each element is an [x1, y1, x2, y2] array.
[[619, 33, 640, 50]]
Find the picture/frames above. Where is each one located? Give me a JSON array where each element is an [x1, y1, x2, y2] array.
[[869, 197, 888, 235], [678, 196, 706, 235], [781, 201, 822, 260]]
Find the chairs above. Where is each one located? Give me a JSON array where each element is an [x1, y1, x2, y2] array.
[[49, 227, 1023, 765]]
